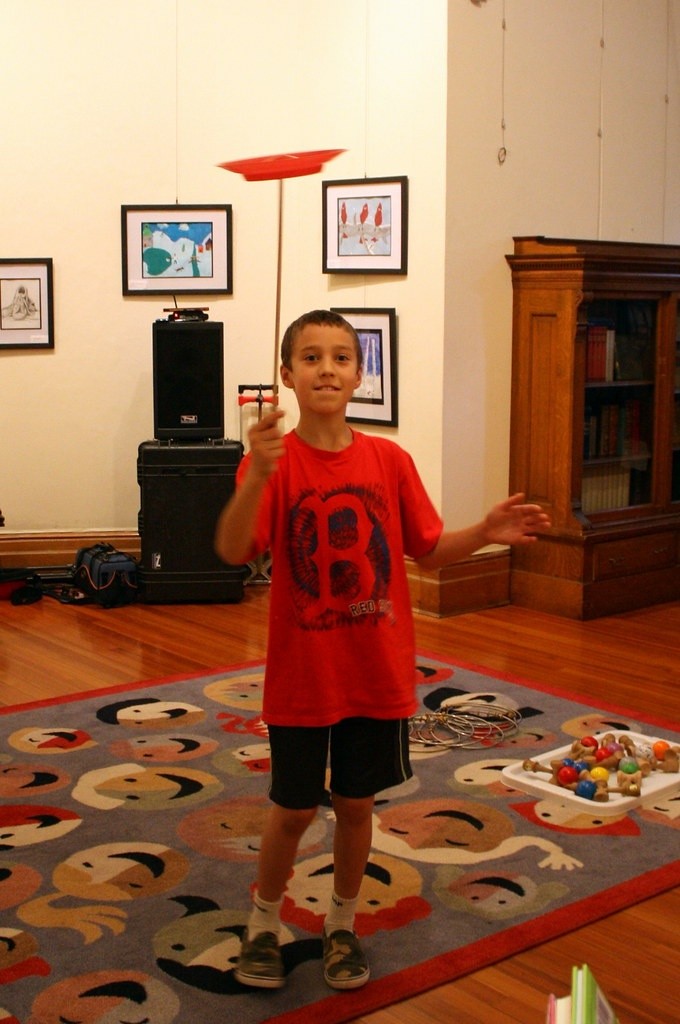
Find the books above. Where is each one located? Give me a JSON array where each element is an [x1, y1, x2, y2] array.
[[583, 399, 638, 458], [588, 328, 614, 382], [581, 463, 629, 511]]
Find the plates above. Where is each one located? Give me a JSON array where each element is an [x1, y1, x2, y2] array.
[[214, 149, 346, 182]]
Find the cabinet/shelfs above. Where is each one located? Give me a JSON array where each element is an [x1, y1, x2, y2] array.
[[504, 236, 680, 623]]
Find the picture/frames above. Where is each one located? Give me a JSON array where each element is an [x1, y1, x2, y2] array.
[[121, 205, 232, 297], [331, 308, 399, 428], [322, 176, 409, 276], [0, 258, 55, 349]]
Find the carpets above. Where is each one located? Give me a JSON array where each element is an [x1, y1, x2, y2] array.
[[1, 651, 680, 1024]]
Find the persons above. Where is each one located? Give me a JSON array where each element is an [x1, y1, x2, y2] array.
[[216, 311, 550, 989]]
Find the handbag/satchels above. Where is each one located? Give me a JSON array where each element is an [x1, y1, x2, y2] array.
[[73, 542, 138, 607]]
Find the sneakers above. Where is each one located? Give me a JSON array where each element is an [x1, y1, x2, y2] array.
[[236, 926, 284, 987], [322, 926, 370, 987]]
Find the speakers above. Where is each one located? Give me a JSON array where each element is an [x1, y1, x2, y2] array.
[[151, 322, 224, 441]]
